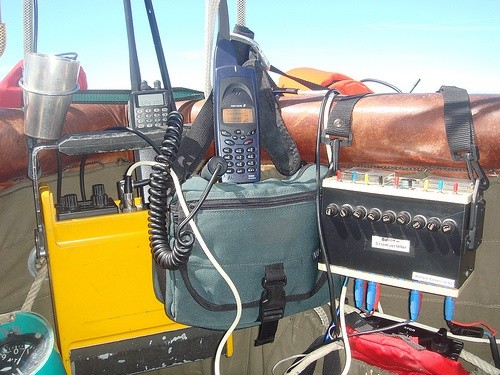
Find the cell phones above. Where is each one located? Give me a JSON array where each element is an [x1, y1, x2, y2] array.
[[212, 62, 260, 186]]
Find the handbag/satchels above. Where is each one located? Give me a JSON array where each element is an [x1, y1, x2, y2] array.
[[151, 162, 342, 347]]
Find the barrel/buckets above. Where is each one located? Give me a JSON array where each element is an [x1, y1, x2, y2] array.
[[0, 311, 67, 375], [18, 50, 80, 140]]
